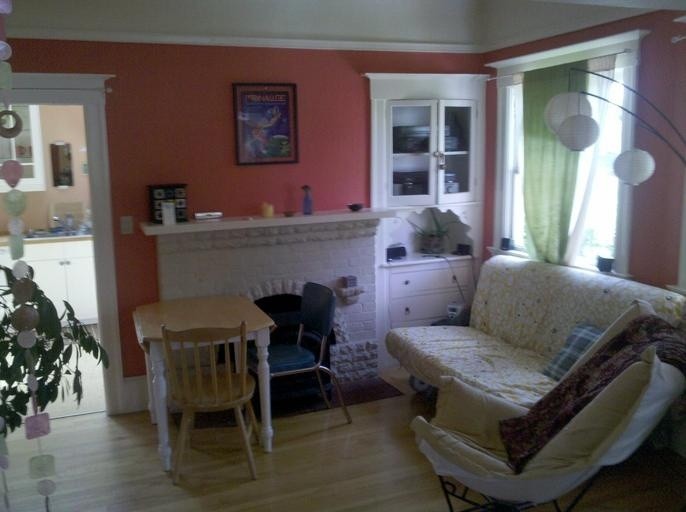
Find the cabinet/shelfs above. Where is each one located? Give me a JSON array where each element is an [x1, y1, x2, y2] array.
[[358, 71, 490, 372]]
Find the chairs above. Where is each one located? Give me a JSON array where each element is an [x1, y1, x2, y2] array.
[[237, 281, 353, 443], [408, 297, 685, 512], [161, 317, 260, 485]]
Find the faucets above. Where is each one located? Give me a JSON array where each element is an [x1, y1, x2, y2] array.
[[52, 211, 75, 230]]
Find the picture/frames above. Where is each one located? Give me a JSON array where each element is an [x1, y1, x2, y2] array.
[[230, 81, 300, 166]]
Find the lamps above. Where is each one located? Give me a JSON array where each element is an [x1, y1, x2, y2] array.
[[543, 66, 686, 187]]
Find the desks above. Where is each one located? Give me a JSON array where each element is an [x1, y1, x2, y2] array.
[[131, 292, 276, 473]]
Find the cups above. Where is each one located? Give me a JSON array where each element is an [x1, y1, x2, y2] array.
[[261, 203, 273, 217]]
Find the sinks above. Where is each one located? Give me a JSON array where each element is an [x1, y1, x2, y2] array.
[[24, 229, 91, 238]]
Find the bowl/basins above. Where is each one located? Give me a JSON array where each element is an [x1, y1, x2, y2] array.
[[346, 202, 364, 212]]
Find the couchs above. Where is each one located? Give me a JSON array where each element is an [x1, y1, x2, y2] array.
[[383, 253, 685, 409]]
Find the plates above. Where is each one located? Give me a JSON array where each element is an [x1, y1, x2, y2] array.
[[280, 210, 298, 217]]
